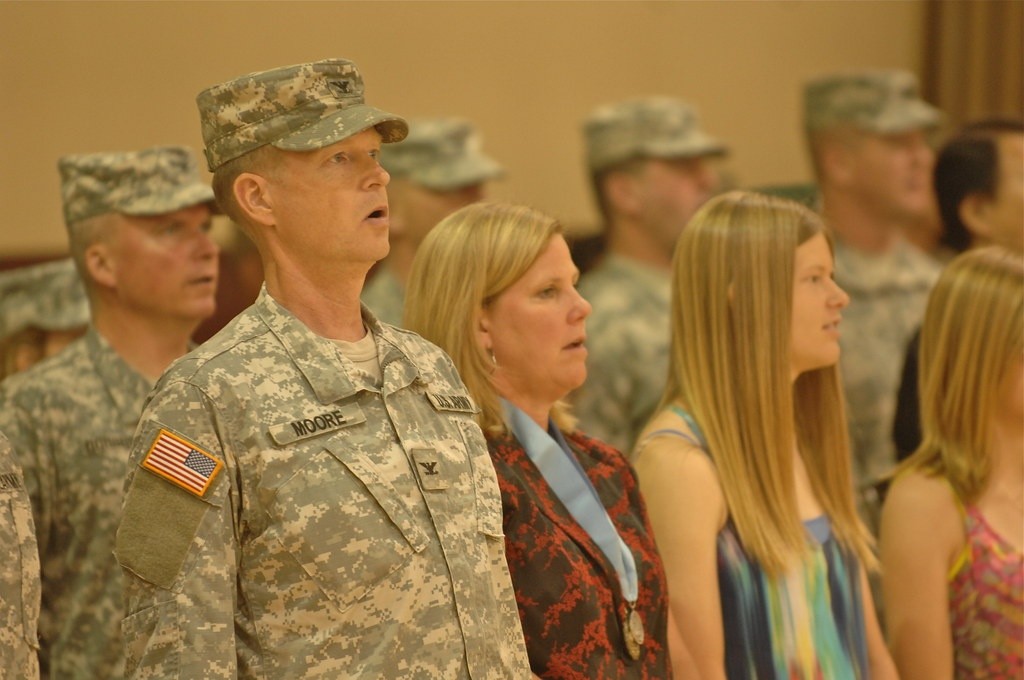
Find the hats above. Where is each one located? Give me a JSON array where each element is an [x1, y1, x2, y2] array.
[[797, 68, 943, 137], [196, 58, 410, 173], [1, 259, 91, 339], [57, 147, 227, 220], [379, 114, 508, 190], [582, 96, 731, 169]]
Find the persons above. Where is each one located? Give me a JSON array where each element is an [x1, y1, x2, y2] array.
[[878, 243, 1024, 680], [114, 58, 533, 679], [403, 201, 673, 679], [0, 145, 226, 680], [631, 191, 899, 679], [0, 70, 1024, 680]]
